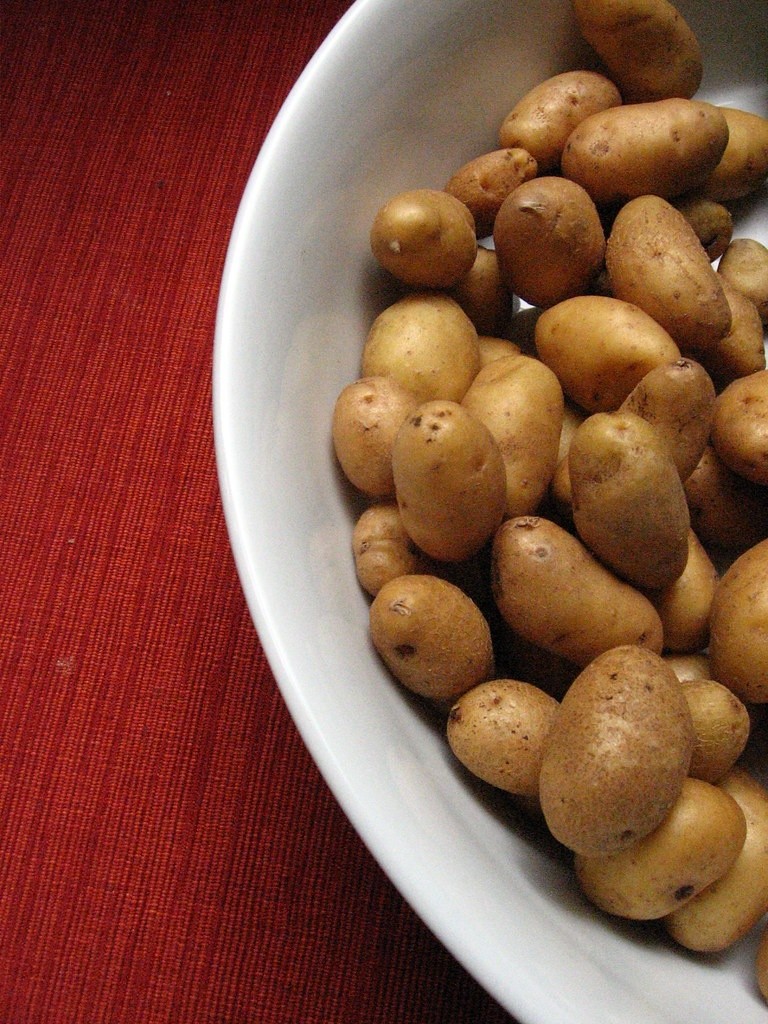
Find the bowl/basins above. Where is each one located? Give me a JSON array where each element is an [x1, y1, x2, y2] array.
[[212, 0, 768, 1024]]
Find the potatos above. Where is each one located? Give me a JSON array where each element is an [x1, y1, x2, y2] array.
[[331, 0, 768, 1007]]
